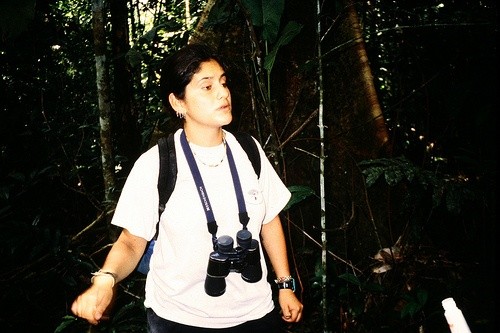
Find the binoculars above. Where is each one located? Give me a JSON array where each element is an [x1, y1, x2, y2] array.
[[204, 229, 263, 297]]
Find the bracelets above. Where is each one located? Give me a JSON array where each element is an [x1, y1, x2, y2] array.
[[274, 274, 296, 291], [90, 269, 118, 288]]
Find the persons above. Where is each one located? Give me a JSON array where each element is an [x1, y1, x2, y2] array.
[[72, 44, 304, 333]]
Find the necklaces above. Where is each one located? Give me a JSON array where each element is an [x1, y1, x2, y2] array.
[[182, 123, 228, 167]]
